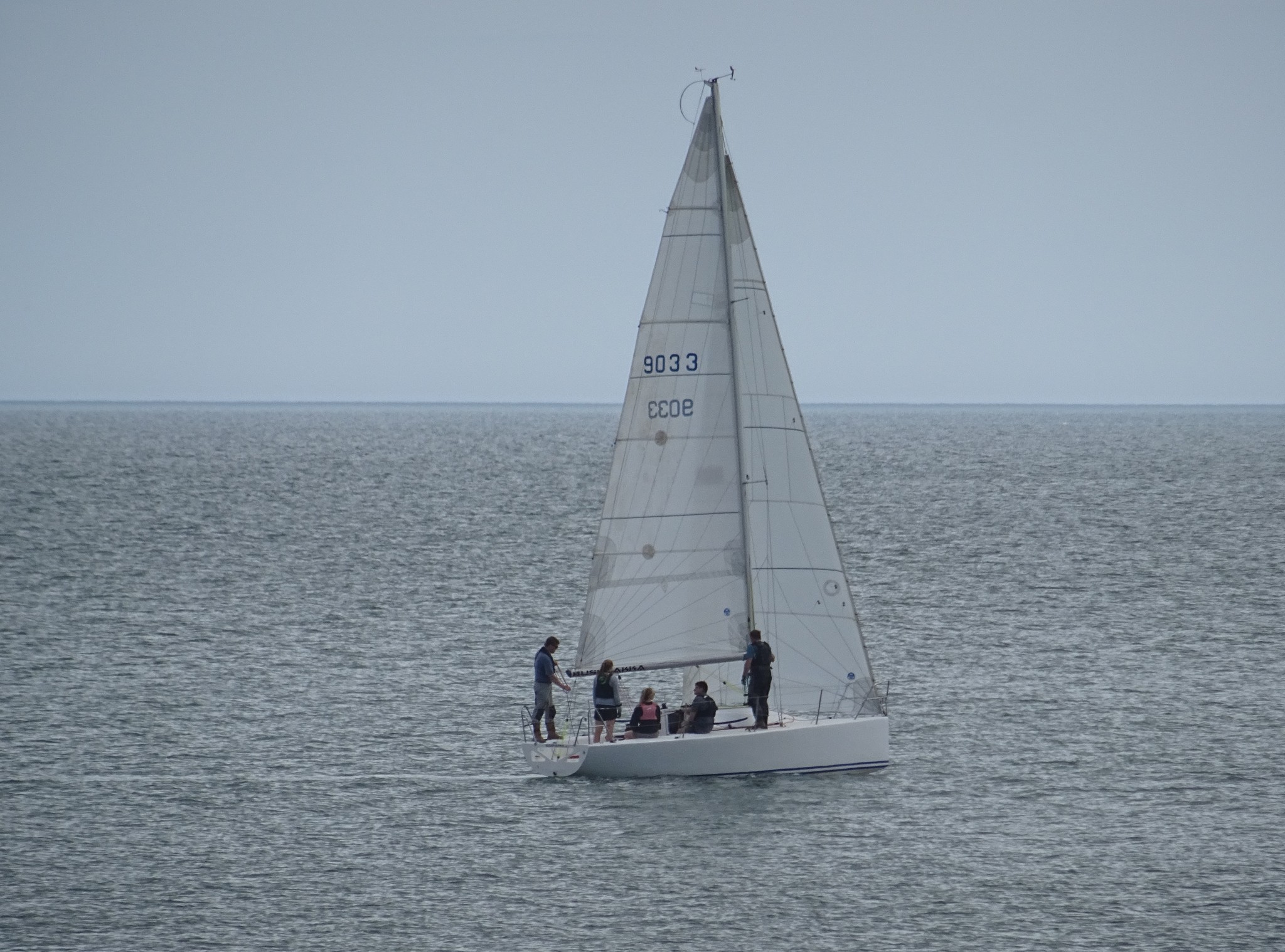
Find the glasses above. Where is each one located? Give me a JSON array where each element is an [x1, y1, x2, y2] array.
[[553, 645, 559, 649]]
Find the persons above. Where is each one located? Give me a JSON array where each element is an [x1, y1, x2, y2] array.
[[677, 680, 717, 734], [741, 630, 775, 730], [531, 636, 571, 743], [624, 688, 660, 739], [591, 659, 622, 743]]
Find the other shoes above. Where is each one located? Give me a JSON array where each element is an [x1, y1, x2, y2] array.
[[750, 720, 768, 730]]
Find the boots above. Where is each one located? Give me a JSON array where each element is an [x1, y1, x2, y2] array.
[[532, 722, 547, 743], [546, 722, 564, 740]]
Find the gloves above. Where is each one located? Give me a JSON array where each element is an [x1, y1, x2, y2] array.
[[741, 675, 746, 686]]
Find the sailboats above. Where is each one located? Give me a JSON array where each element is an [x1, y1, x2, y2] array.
[[519, 63, 891, 783]]
